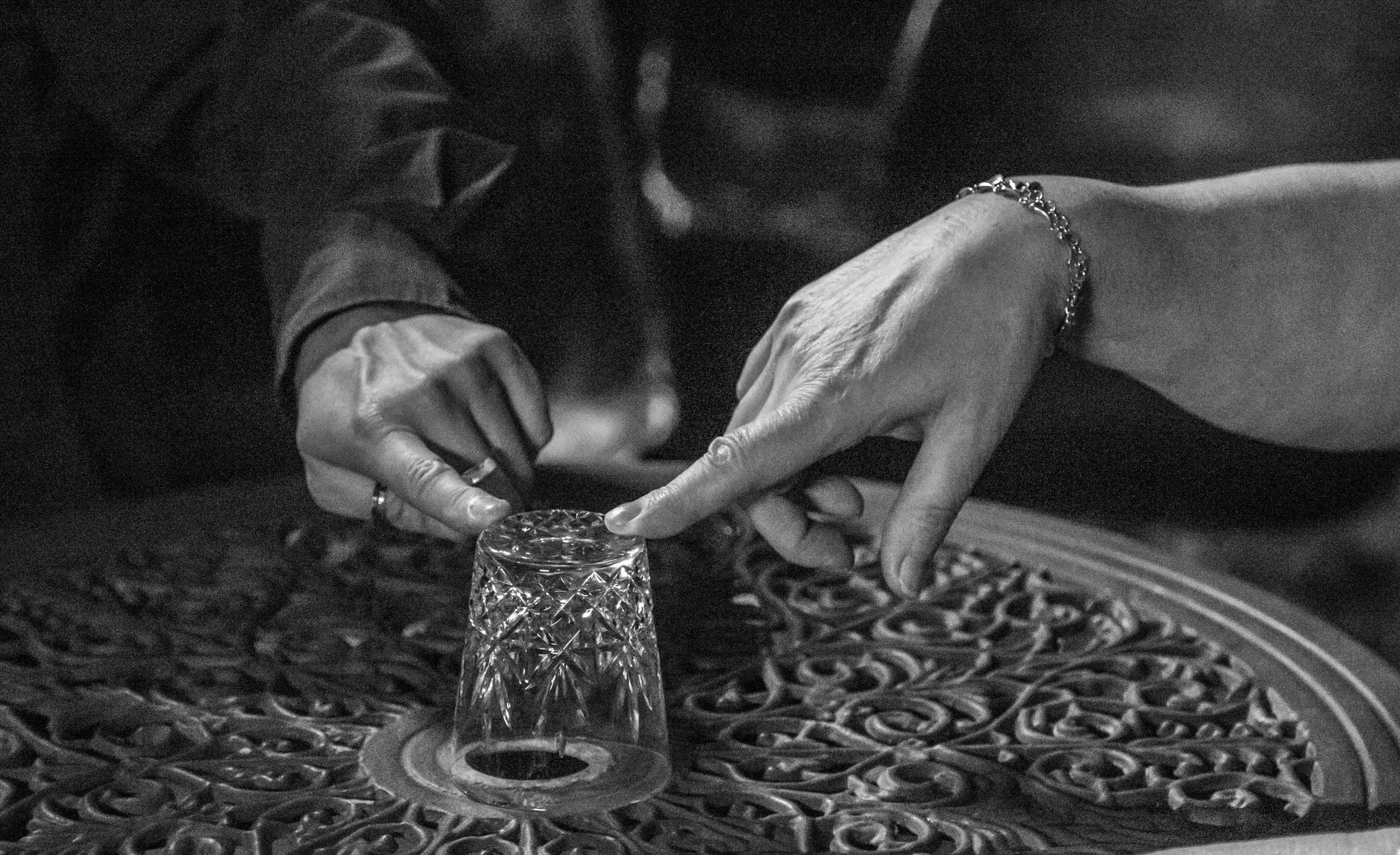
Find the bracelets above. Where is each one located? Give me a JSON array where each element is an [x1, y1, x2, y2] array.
[[952, 174, 1087, 352]]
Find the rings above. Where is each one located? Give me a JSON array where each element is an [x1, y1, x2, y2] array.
[[368, 481, 389, 529], [462, 457, 499, 483]]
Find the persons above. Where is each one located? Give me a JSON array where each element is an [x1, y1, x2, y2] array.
[[604, 160, 1400, 603], [0, 0, 553, 543]]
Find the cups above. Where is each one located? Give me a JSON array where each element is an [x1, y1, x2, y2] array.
[[448, 508, 675, 820]]
